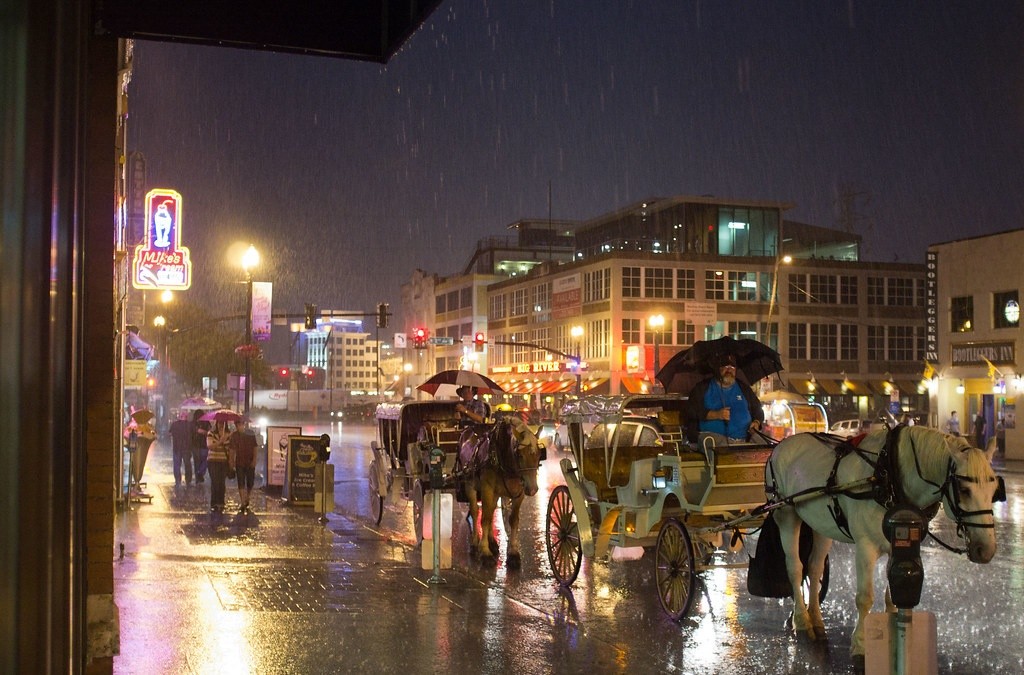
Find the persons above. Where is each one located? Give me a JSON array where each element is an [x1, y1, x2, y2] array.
[[206, 420, 234, 512], [685, 349, 765, 445], [168, 412, 194, 488], [972, 411, 988, 450], [229, 421, 258, 513], [946, 411, 962, 437], [996, 418, 1007, 458], [454, 386, 486, 424], [190, 409, 212, 484]]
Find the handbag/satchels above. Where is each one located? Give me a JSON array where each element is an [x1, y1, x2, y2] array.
[[227, 464, 236, 479]]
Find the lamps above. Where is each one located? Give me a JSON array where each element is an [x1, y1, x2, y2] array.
[[992, 377, 1002, 394], [956, 379, 965, 394]]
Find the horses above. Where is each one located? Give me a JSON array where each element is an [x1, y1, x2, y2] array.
[[457, 416, 544, 568], [765, 425, 998, 675]]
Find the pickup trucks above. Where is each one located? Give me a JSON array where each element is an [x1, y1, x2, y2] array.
[[828, 418, 874, 440]]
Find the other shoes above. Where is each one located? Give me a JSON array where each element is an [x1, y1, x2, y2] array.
[[241, 501, 248, 509], [195, 473, 204, 482]]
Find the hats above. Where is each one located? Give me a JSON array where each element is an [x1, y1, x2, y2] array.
[[719, 352, 737, 367], [456, 385, 478, 397]]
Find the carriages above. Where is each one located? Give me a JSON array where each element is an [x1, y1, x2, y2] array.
[[544, 390, 1006, 674], [366, 395, 544, 572]]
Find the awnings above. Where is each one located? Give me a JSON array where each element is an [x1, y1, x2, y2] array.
[[788, 379, 930, 397], [126, 329, 151, 360], [498, 378, 611, 392]]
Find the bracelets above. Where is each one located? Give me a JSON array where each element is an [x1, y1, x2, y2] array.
[[464, 409, 469, 415]]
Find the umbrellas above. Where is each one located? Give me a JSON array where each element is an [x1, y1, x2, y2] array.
[[196, 409, 252, 436], [654, 335, 786, 398], [177, 396, 223, 409], [416, 368, 506, 401]]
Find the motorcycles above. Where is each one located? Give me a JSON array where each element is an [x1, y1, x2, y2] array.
[[757, 398, 829, 442]]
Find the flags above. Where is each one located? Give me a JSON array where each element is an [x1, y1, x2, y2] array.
[[986, 361, 995, 382], [924, 361, 934, 379]]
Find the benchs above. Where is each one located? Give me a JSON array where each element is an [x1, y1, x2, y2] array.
[[658, 445, 708, 490], [579, 445, 678, 502]]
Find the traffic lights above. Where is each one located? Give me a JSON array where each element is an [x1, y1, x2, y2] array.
[[474, 331, 484, 352], [415, 327, 427, 349]]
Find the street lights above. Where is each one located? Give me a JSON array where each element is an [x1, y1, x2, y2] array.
[[240, 242, 262, 432], [763, 252, 793, 350], [151, 290, 174, 447], [570, 326, 584, 399], [648, 314, 664, 395]]
